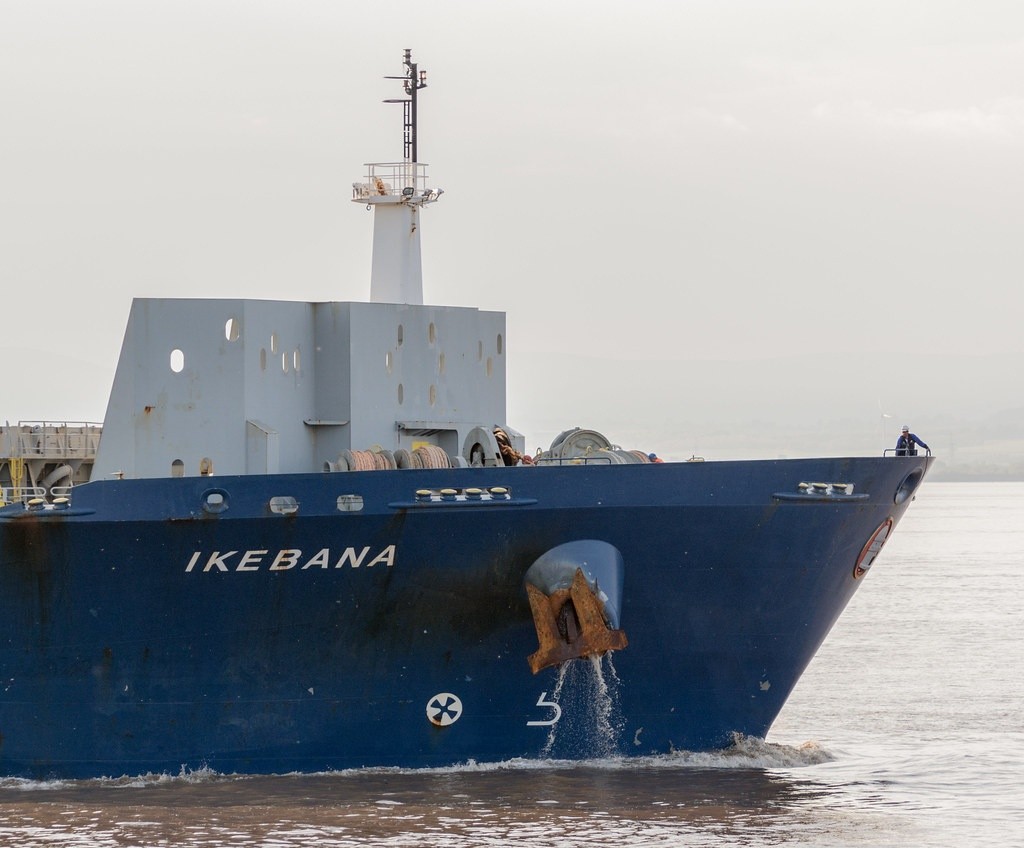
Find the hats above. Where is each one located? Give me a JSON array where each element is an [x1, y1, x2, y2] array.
[[902, 425, 909, 432], [648, 453, 656, 459]]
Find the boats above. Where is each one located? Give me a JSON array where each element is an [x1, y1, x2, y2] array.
[[1, 48, 937, 779]]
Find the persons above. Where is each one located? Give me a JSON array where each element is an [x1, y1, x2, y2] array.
[[895, 425, 931, 456], [649, 453, 664, 463]]
